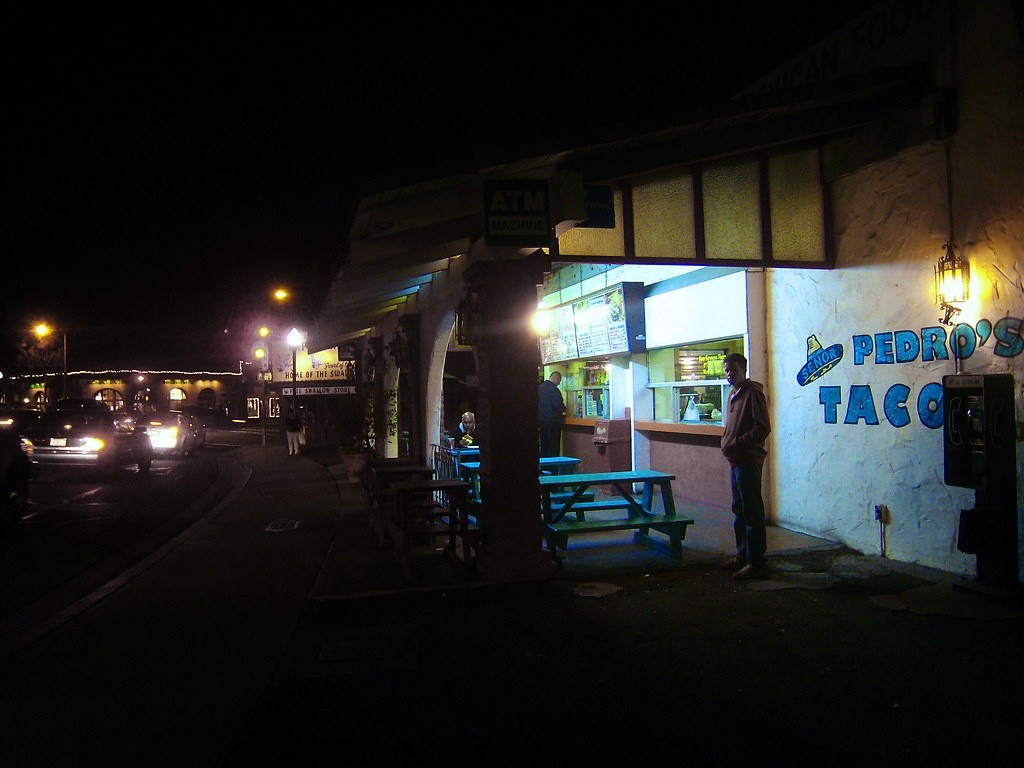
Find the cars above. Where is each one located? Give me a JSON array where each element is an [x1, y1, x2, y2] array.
[[0, 399, 207, 526]]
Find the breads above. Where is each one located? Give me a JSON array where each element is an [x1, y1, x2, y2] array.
[[464, 434, 473, 440]]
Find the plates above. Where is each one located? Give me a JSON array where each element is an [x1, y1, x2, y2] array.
[[467, 446, 479, 449]]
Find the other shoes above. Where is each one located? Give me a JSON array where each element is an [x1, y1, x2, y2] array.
[[725, 556, 748, 570], [733, 562, 769, 579], [295, 449, 297, 454], [289, 451, 292, 455]]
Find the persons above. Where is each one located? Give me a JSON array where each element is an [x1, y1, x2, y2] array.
[[721, 354, 771, 580], [451, 411, 480, 483], [538, 371, 567, 492], [285, 408, 300, 456]]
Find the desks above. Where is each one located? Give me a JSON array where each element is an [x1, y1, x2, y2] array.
[[372, 444, 677, 587]]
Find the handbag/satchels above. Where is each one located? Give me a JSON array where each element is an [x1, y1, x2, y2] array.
[[298, 426, 306, 445]]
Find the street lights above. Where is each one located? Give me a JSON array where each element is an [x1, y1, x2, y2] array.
[[32, 322, 68, 400], [284, 327, 302, 413], [254, 347, 268, 448]]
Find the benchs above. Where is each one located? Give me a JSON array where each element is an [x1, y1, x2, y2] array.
[[539, 490, 696, 569]]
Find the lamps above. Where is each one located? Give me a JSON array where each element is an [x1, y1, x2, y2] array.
[[454, 282, 484, 346], [932, 129, 971, 328]]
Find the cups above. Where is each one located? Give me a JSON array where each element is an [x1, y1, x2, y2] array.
[[448, 438, 455, 450]]
